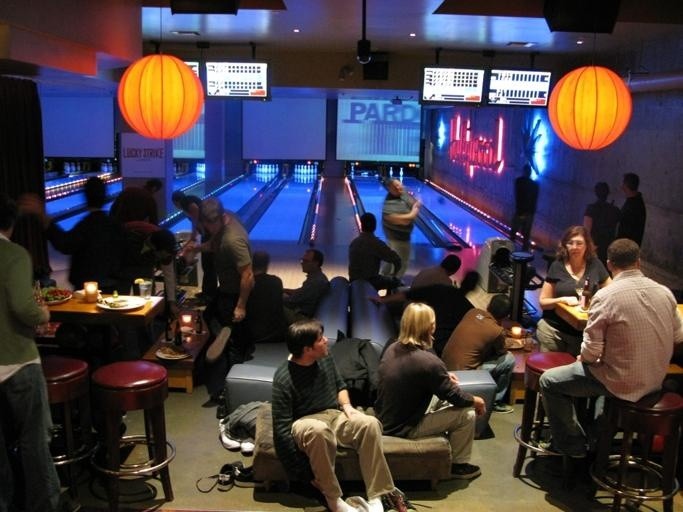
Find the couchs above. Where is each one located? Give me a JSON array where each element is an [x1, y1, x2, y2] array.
[[352, 273, 500, 442], [226, 277, 346, 435]]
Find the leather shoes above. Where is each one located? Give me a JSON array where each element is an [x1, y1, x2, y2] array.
[[217, 390, 228, 419], [203, 393, 218, 407]]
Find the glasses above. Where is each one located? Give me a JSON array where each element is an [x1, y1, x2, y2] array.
[[565, 240, 586, 246], [299, 257, 314, 265]]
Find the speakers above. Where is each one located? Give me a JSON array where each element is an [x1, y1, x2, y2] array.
[[362, 49, 390, 81]]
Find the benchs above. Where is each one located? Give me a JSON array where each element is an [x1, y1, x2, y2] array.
[[254, 405, 454, 497]]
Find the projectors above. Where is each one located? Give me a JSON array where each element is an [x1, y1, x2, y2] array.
[[392, 98, 402, 104]]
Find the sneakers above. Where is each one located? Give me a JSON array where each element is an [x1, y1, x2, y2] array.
[[380, 495, 397, 511], [217, 461, 244, 491], [451, 462, 480, 480], [538, 439, 586, 458], [491, 403, 512, 413], [389, 488, 415, 511], [235, 467, 272, 487]]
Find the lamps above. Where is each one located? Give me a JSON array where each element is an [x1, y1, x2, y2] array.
[[115, 55, 204, 139], [546, 65, 632, 149]]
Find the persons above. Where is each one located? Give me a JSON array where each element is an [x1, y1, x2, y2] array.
[[616, 172, 645, 249], [539, 238, 682, 455], [124, 221, 179, 313], [284, 249, 331, 320], [489, 247, 514, 286], [1, 197, 61, 511], [58, 176, 135, 295], [584, 183, 619, 278], [347, 214, 404, 290], [12, 190, 56, 290], [536, 226, 613, 352], [377, 302, 487, 479], [189, 200, 253, 420], [171, 191, 203, 241], [443, 295, 516, 413], [368, 270, 481, 360], [510, 164, 539, 253], [246, 252, 283, 344], [110, 180, 162, 225], [382, 180, 421, 286], [412, 255, 462, 286], [272, 320, 407, 511]]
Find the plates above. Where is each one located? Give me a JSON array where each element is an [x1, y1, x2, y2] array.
[[95, 295, 147, 310], [509, 339, 524, 348], [42, 293, 72, 305], [154, 346, 192, 359]]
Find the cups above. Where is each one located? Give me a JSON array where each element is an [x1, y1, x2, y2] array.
[[139, 281, 152, 300], [83, 282, 98, 303]]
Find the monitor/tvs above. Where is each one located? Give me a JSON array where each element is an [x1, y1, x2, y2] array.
[[418, 65, 485, 106], [204, 60, 272, 103], [182, 60, 203, 83], [486, 67, 552, 109]]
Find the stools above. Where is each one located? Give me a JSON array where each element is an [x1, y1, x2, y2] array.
[[591, 390, 681, 511], [43, 359, 91, 509], [514, 352, 575, 477], [92, 363, 176, 512]]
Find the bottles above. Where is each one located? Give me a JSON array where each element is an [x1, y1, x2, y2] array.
[[581, 280, 590, 311], [175, 320, 182, 346], [166, 315, 173, 341], [196, 309, 202, 337], [34, 279, 50, 338]]
